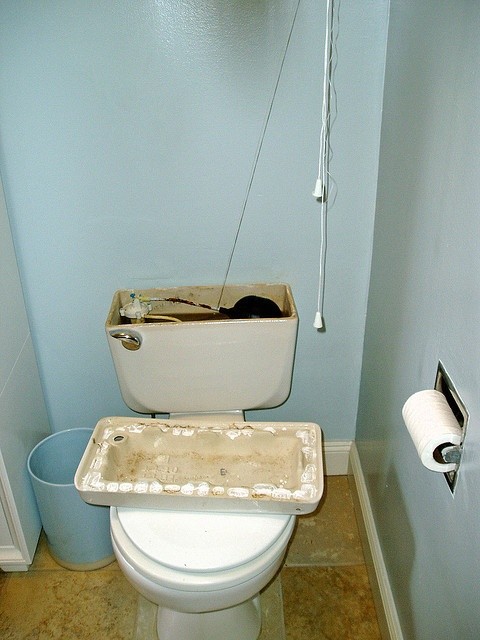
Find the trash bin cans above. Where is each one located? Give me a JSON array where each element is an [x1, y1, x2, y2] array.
[[26, 427, 117, 571]]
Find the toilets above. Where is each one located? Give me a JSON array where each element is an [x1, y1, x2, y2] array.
[[74, 280, 325, 639]]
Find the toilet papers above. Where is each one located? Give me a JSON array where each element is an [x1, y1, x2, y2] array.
[[401, 358, 468, 501]]
